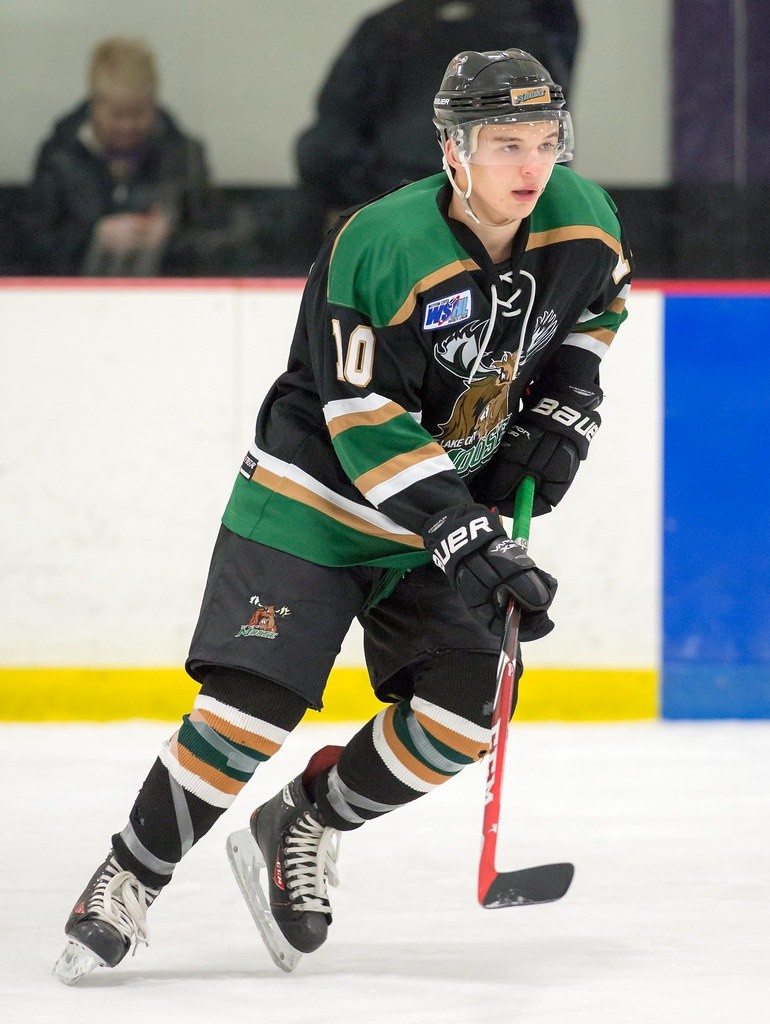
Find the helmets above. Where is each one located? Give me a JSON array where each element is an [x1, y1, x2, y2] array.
[[435, 47, 575, 163]]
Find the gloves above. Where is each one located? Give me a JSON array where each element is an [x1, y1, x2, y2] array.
[[473, 373, 604, 519], [422, 503, 558, 641]]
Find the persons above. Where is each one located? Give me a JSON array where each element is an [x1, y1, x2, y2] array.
[[60, 46, 633, 977], [27, 41, 210, 277], [293, 1, 579, 267]]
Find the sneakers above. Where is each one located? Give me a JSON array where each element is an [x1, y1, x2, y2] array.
[[226, 746, 348, 974], [51, 850, 162, 985]]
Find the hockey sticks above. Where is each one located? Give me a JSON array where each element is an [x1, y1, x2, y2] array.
[[470, 469, 576, 909]]
